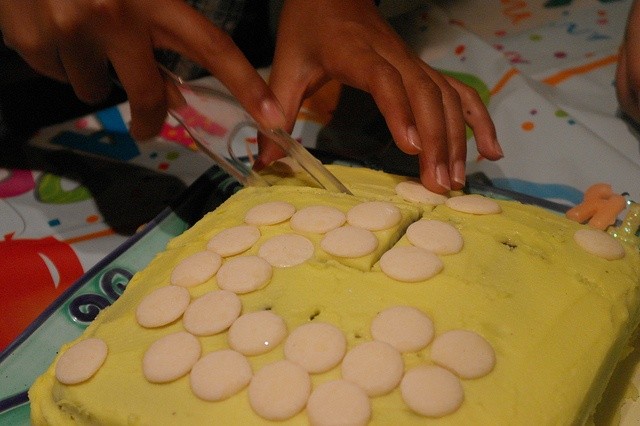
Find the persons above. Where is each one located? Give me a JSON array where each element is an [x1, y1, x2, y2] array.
[[613, 1, 640, 128], [0, 0, 504, 194]]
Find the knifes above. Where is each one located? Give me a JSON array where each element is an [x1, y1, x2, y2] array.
[[68, 32, 358, 197]]
